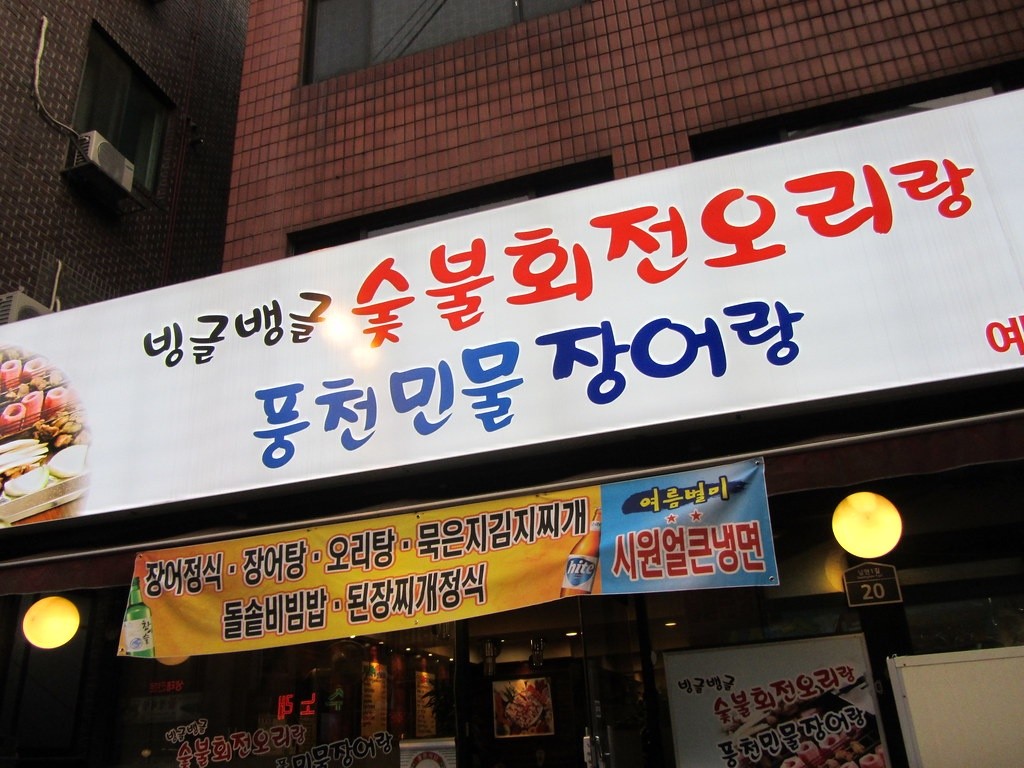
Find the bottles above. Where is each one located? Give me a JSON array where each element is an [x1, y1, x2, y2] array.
[[560, 508, 602, 596], [123, 576, 155, 657]]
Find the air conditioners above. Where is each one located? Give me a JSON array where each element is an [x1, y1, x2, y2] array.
[[69, 130, 136, 194]]
